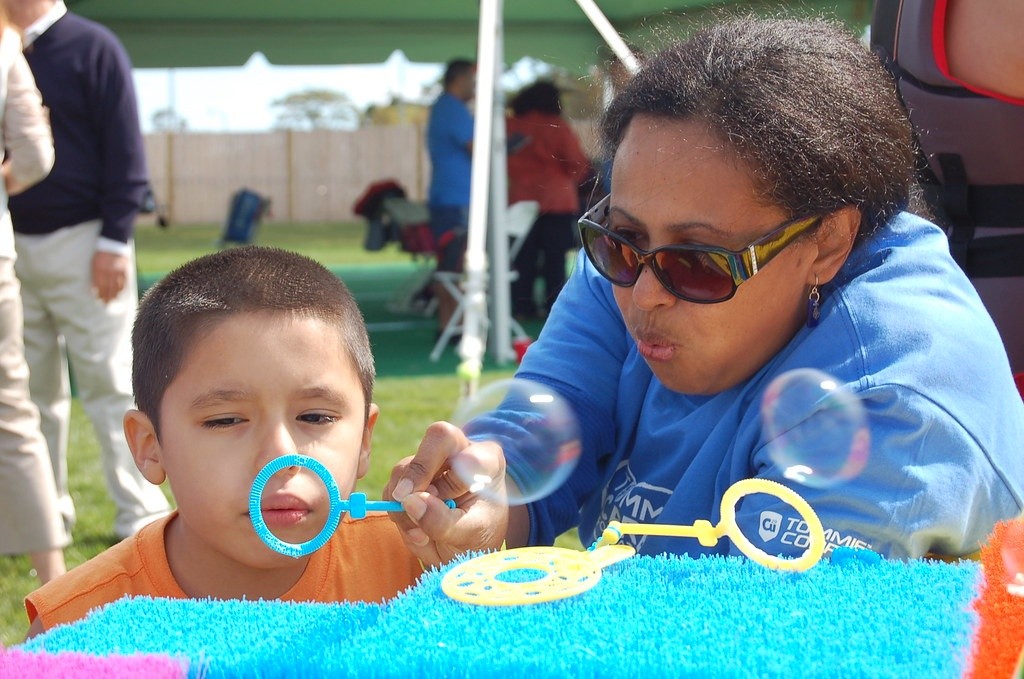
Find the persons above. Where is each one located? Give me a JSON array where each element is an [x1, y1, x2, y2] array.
[[506, 80, 591, 322], [381, 18, 1024, 568], [604, 45, 646, 195], [0, 0, 171, 584], [0, 0, 70, 584], [424, 60, 477, 344], [22, 245, 427, 643]]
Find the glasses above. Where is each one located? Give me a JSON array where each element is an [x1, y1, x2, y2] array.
[[578, 192, 822, 304]]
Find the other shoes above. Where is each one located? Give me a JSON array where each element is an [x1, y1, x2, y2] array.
[[435, 328, 461, 345]]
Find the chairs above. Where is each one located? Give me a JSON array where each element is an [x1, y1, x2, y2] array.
[[431, 199, 541, 358]]
